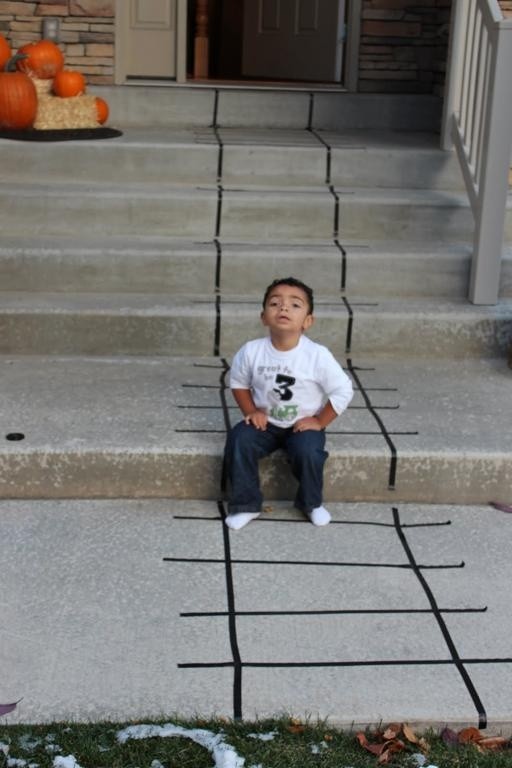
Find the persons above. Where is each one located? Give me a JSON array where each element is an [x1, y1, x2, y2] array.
[[224, 278, 354, 530]]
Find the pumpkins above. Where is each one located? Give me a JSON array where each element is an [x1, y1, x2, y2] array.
[[0, 31, 108, 132]]
